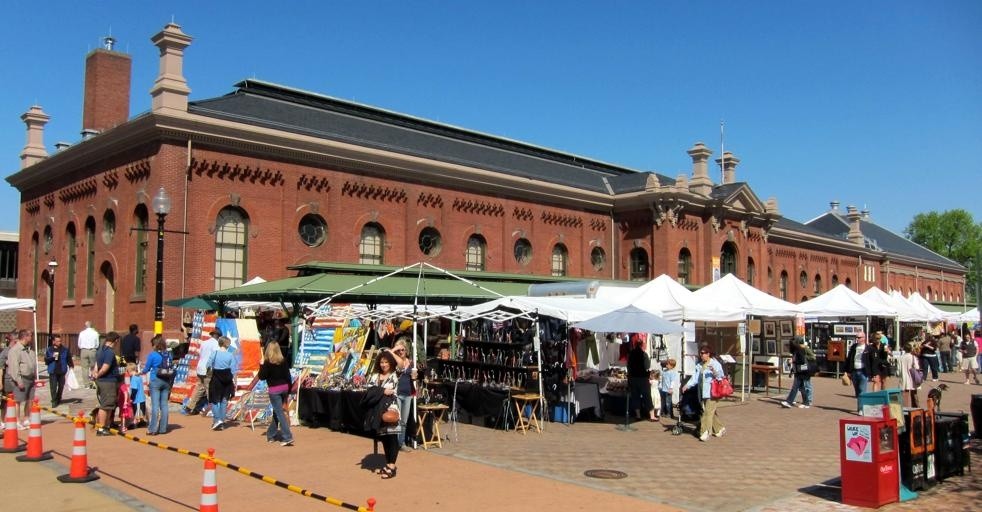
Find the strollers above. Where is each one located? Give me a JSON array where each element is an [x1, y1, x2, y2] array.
[[671, 373, 702, 437]]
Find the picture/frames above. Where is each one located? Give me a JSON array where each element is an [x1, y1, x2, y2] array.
[[751, 319, 794, 375], [832, 323, 865, 337]]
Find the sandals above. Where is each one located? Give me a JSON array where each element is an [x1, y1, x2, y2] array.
[[376, 465, 398, 480]]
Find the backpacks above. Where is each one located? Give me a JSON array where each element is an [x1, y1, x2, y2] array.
[[154, 350, 177, 382]]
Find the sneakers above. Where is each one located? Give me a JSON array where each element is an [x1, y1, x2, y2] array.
[[857, 409, 864, 416], [699, 426, 727, 442], [780, 400, 794, 410], [964, 377, 980, 384], [401, 443, 411, 452], [0, 419, 30, 431], [209, 419, 225, 432], [281, 438, 295, 447], [93, 419, 170, 437], [798, 403, 811, 410]]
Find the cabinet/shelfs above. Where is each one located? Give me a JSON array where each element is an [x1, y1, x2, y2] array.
[[427, 318, 537, 431]]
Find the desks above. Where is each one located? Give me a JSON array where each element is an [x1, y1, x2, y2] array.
[[577, 367, 632, 428], [570, 381, 601, 424], [752, 364, 781, 396], [512, 394, 545, 435], [298, 382, 376, 439], [417, 403, 449, 450]]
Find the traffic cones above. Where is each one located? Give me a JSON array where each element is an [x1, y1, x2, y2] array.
[[56, 410, 101, 484], [17, 398, 53, 462], [198, 448, 220, 512], [0, 394, 27, 452]]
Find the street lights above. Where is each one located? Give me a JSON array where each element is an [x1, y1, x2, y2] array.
[[130, 186, 191, 336], [44, 256, 58, 364]]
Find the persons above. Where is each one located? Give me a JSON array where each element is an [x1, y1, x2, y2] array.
[[391, 337, 418, 453], [88, 323, 176, 436], [627, 338, 726, 442], [358, 348, 405, 479], [780, 337, 817, 409], [8, 327, 37, 431], [0, 330, 23, 430], [43, 334, 74, 409], [182, 328, 223, 416], [205, 336, 238, 431], [842, 330, 982, 416], [258, 340, 295, 447], [77, 320, 100, 389]]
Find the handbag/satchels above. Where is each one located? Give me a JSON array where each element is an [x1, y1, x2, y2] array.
[[711, 367, 735, 400], [840, 371, 852, 386], [909, 354, 923, 388], [380, 408, 400, 425]]
